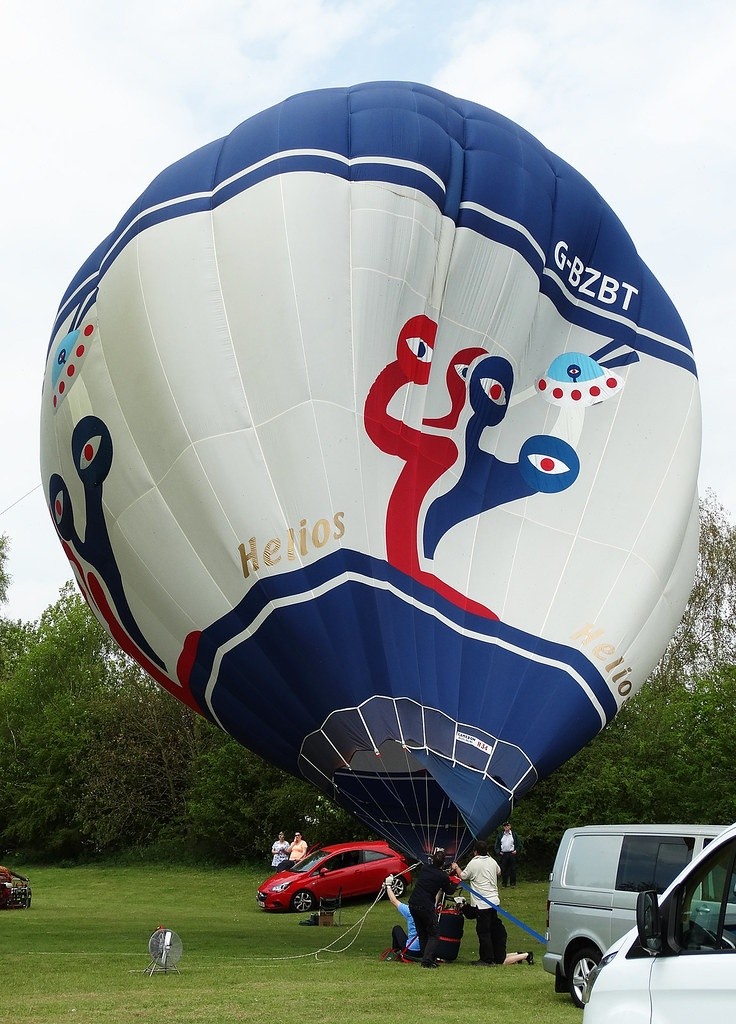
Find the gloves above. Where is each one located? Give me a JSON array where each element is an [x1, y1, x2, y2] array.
[[385, 874, 393, 885]]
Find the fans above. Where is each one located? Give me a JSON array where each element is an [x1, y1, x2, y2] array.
[[143, 928, 183, 977]]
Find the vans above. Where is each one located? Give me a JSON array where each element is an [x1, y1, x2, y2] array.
[[542, 823, 736, 1009], [582, 822, 736, 1024]]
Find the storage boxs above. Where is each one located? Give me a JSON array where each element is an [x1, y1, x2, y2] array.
[[318, 910, 336, 926]]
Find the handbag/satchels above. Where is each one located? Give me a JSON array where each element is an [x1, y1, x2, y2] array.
[[380, 948, 402, 962]]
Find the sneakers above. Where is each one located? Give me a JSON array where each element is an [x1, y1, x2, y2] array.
[[421, 957, 440, 969], [526, 951, 535, 965], [501, 886, 505, 889], [510, 885, 516, 889]]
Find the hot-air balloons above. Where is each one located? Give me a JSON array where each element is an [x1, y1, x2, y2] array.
[[36, 77, 702, 961]]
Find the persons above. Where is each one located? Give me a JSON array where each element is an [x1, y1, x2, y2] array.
[[495, 821, 520, 889], [271, 831, 289, 867], [287, 831, 307, 864], [386, 841, 535, 969]]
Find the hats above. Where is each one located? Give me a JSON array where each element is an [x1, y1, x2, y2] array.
[[502, 821, 511, 827], [279, 831, 284, 835]]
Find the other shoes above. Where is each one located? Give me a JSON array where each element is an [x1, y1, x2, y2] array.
[[517, 951, 523, 964]]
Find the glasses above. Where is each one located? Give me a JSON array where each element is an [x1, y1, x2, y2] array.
[[503, 824, 509, 826], [295, 835, 300, 837]]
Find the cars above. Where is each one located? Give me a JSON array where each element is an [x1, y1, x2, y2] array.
[[256, 838, 412, 912]]
